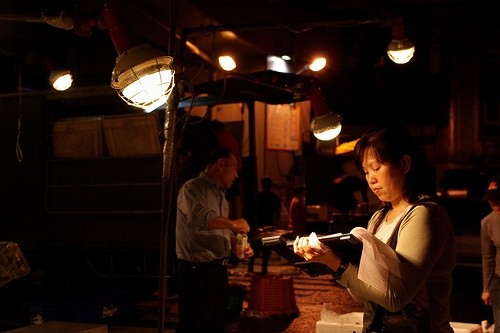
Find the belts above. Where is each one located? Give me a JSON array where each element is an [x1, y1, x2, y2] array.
[[175, 259, 225, 271]]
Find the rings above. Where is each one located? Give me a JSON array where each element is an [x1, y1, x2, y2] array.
[[302, 251, 304, 255]]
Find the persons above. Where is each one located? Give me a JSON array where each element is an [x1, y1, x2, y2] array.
[[293, 129, 455, 333], [480, 178, 500, 326], [173, 147, 250, 333], [248, 177, 282, 272]]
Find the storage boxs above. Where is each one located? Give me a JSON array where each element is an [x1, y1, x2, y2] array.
[[316, 311, 481, 333]]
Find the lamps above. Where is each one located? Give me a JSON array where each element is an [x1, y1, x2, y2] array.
[[307, 87, 343, 141], [386, 17, 415, 64], [102, 2, 176, 114], [43, 57, 74, 92]]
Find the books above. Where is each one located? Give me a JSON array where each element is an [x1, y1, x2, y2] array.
[[286, 226, 402, 294]]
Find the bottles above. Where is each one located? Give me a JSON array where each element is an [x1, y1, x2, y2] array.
[[236, 231, 248, 259]]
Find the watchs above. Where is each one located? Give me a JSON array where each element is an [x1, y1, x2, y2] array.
[[328, 259, 349, 280]]
